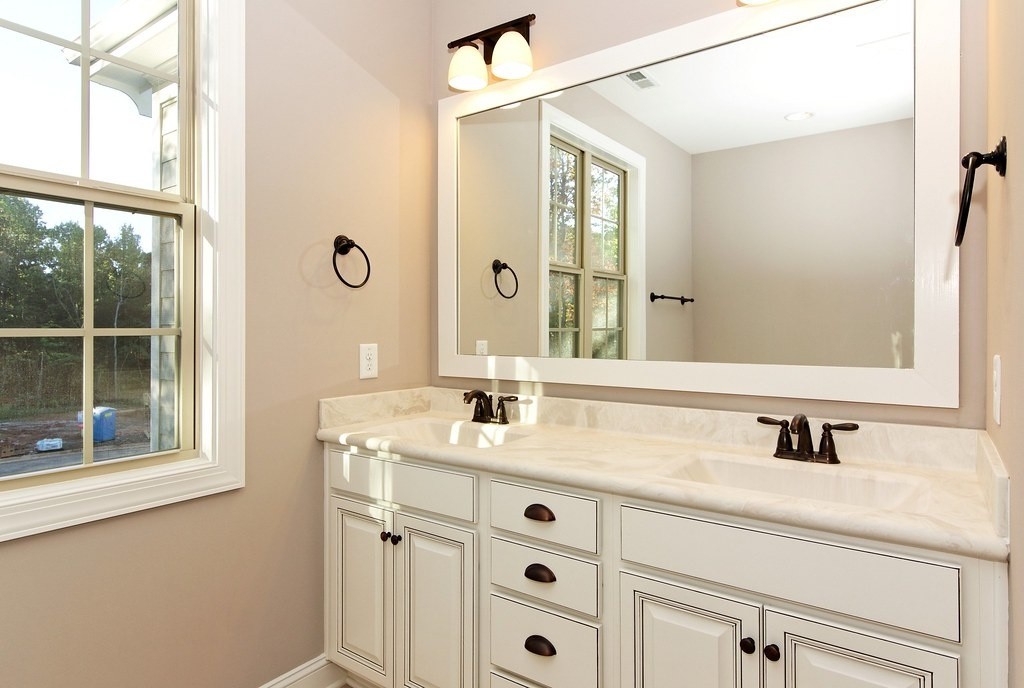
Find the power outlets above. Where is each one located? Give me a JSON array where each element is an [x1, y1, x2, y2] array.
[[359, 343, 380, 379], [475, 340, 488, 356]]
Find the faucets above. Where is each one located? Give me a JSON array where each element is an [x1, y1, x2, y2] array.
[[788, 413, 818, 460], [463, 389, 494, 422]]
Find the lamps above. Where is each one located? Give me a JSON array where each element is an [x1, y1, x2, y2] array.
[[446, 13, 536, 90]]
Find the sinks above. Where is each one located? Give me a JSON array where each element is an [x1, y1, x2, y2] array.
[[371, 421, 529, 449], [657, 457, 926, 514]]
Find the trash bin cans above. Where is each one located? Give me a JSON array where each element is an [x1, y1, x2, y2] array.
[[93, 405, 116, 442]]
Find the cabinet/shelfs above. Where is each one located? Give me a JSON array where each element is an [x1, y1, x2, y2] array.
[[316, 442, 1011, 688]]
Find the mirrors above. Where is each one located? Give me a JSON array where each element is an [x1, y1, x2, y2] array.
[[457, 0, 916, 368]]
